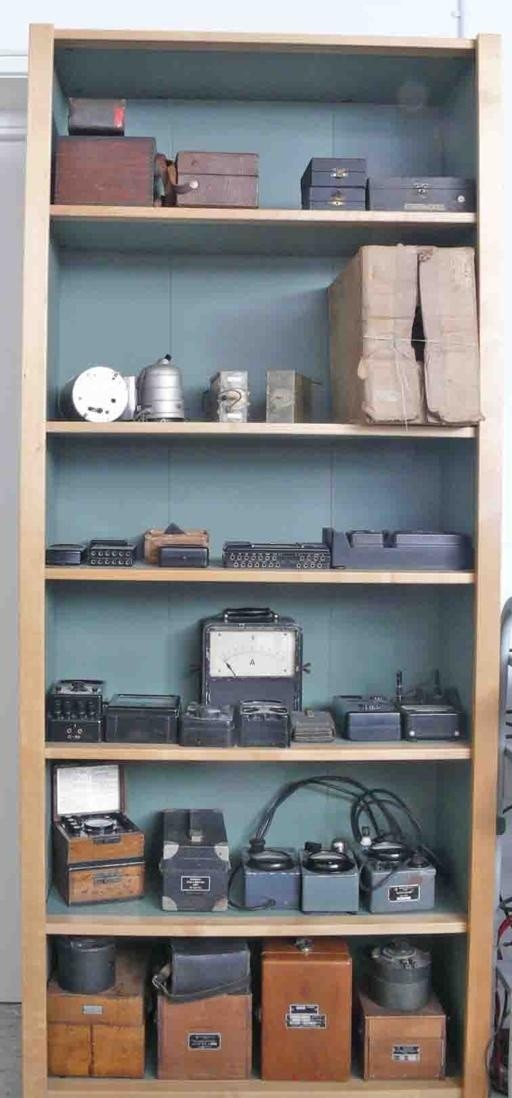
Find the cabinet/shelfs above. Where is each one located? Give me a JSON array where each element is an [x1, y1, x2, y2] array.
[[14, 22, 507, 1098]]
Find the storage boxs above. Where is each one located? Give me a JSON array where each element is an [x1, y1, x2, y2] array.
[[52, 760, 233, 911], [327, 242, 479, 427]]
